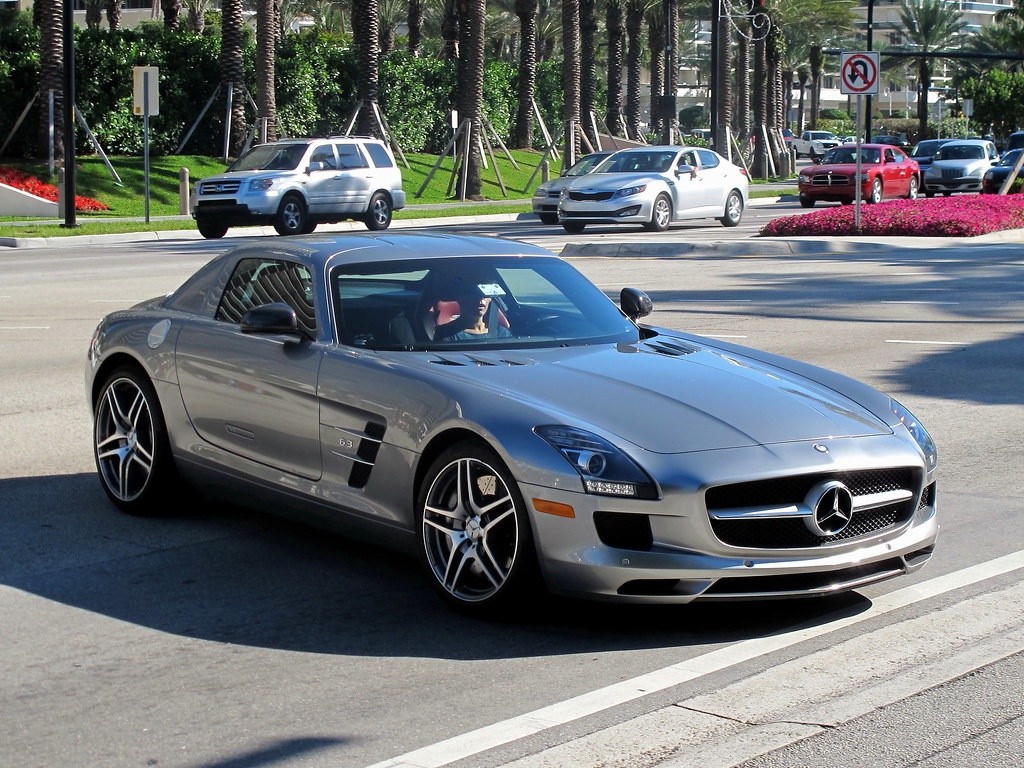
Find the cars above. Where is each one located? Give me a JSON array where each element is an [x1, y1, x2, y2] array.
[[85, 230, 940, 619], [798, 144, 921, 207], [556, 145, 749, 233], [533, 149, 672, 225], [752, 128, 1024, 198]]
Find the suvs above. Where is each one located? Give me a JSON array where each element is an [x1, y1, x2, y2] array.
[[189, 135, 406, 235]]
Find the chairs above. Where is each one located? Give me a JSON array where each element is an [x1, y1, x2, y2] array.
[[415, 267, 513, 343]]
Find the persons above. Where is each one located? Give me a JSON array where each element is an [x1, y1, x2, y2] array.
[[673, 154, 697, 179], [972, 148, 981, 159], [297, 150, 325, 169], [863, 150, 877, 164], [432, 270, 514, 343]]
